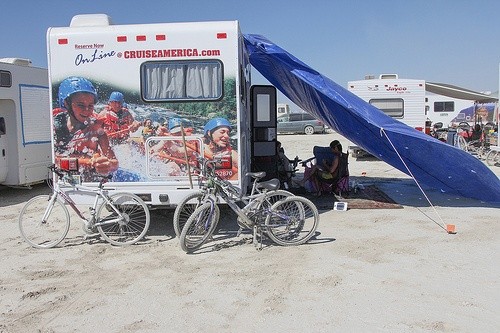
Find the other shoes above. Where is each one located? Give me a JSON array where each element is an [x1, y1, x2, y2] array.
[[293, 180, 304, 187], [311, 190, 321, 198]]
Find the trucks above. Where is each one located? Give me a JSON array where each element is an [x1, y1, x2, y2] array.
[[47, 12, 279, 215], [348, 73, 426, 159], [0, 57, 52, 190]]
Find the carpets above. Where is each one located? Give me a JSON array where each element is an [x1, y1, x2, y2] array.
[[279, 185, 404, 209]]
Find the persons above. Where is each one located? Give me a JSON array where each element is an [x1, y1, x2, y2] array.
[[471, 124, 483, 146], [272, 138, 290, 184], [292, 140, 348, 195], [53, 76, 239, 181]]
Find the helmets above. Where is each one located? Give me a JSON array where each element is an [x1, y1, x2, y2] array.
[[203, 117, 232, 137], [109, 92, 124, 101], [59, 77, 97, 109], [168, 118, 184, 133]]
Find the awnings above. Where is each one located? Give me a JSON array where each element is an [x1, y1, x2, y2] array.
[[426, 82, 499, 104]]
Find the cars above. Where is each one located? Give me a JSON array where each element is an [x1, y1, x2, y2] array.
[[173, 157, 305, 245], [277, 112, 325, 135]]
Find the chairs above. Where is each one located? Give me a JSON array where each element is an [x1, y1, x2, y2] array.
[[277, 146, 350, 196]]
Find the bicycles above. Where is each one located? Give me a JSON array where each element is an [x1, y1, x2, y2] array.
[[18, 162, 151, 249], [432, 122, 500, 167], [179, 173, 319, 253]]
